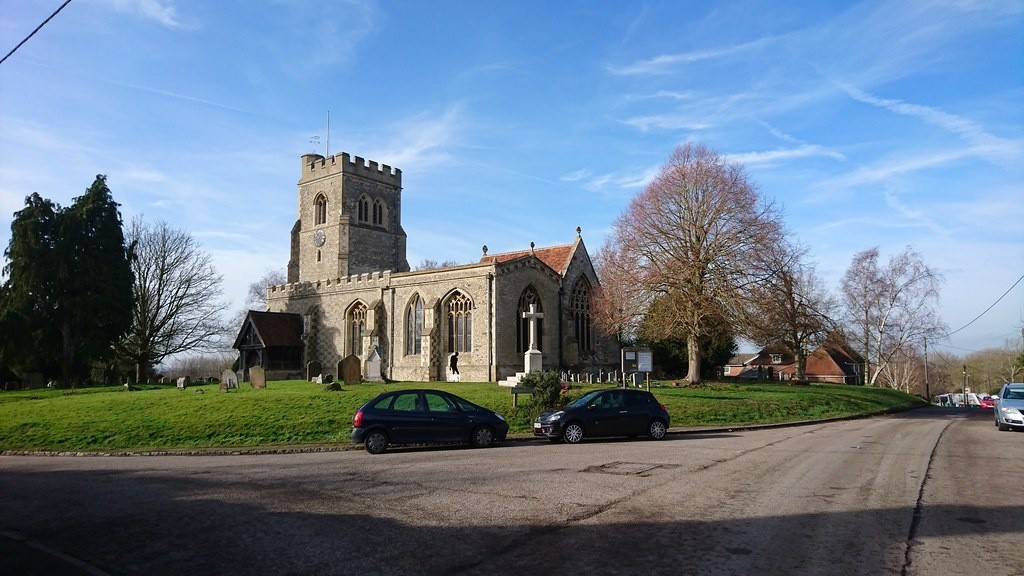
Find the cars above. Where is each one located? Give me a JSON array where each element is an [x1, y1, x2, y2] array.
[[351, 387, 510, 454], [979, 395, 995, 409], [993, 382, 1024, 431], [533, 388, 672, 445]]
